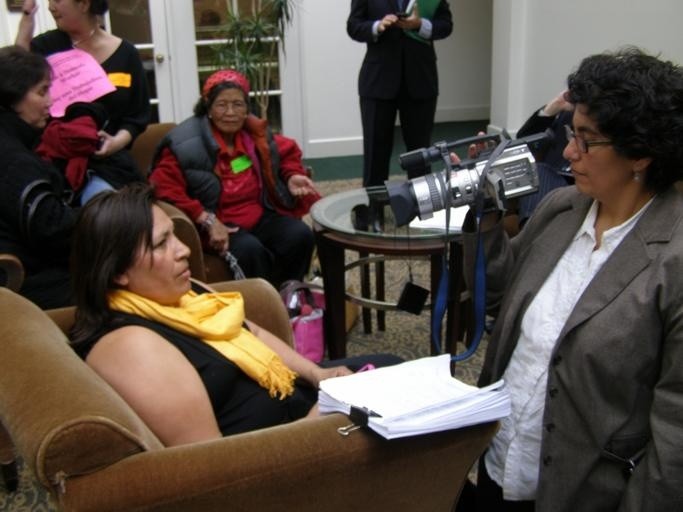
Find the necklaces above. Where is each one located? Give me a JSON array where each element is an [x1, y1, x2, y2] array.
[[72, 22, 99, 47]]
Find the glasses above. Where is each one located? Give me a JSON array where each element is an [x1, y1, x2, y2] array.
[[564, 125, 612, 156]]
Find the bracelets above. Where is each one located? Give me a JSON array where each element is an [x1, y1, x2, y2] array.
[[200, 211, 217, 235]]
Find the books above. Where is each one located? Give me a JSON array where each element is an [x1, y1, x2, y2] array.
[[401, 0, 441, 46]]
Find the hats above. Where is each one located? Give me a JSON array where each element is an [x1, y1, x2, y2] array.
[[203, 70, 250, 100]]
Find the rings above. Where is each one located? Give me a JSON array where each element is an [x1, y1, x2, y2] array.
[[214, 245, 218, 249], [25, 11, 29, 15]]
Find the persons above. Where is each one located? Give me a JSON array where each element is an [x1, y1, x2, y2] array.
[[14, 0, 150, 206], [62, 181, 408, 449], [515, 88, 575, 229], [0, 45, 82, 310], [345, 0, 453, 186], [450, 44, 683, 512], [145, 68, 317, 294]]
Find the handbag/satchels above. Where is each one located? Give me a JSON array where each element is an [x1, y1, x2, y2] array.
[[276, 291, 326, 365]]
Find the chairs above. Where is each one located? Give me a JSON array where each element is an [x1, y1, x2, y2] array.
[[128, 122, 181, 178], [0, 284, 499, 512]]
